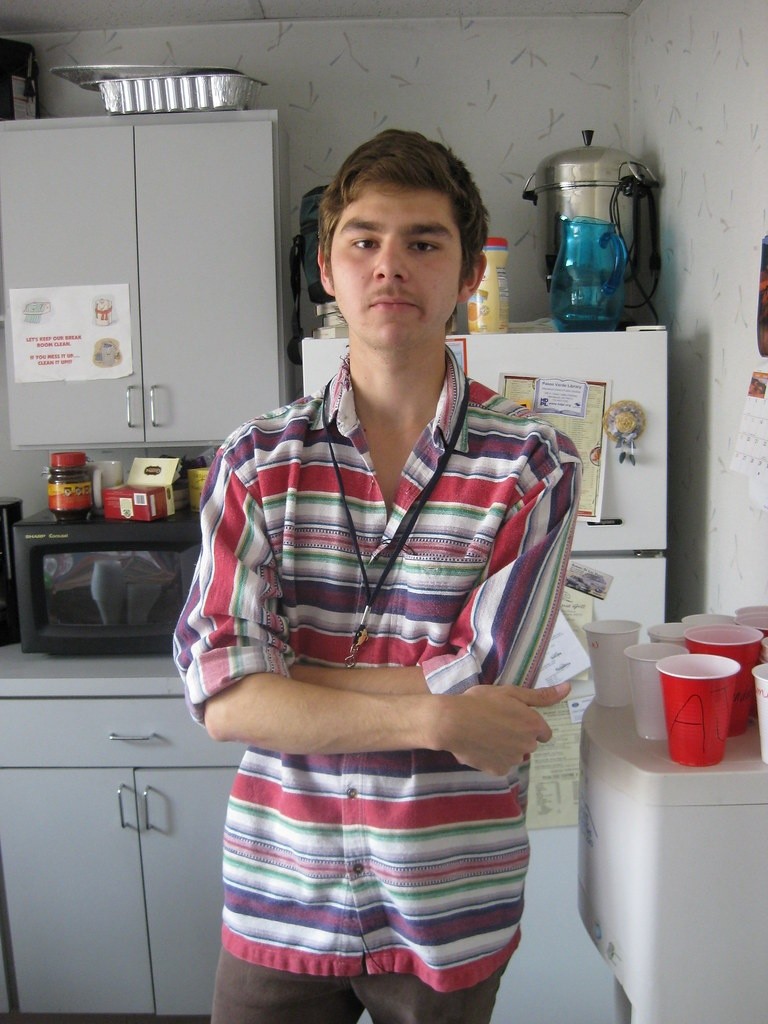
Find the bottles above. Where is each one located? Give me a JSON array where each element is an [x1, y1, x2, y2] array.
[[466, 236, 511, 335]]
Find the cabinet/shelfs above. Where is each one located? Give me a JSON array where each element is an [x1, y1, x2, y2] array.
[[0, 697, 249, 1019], [0, 107, 289, 454]]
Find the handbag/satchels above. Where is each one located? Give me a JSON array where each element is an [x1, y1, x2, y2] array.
[[287, 185, 336, 366]]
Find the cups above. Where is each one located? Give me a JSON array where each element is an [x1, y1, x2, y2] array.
[[85, 460, 125, 516], [750, 663, 768, 766], [646, 603, 768, 665], [683, 625, 764, 736], [622, 642, 690, 743], [655, 652, 742, 768], [582, 620, 642, 710]]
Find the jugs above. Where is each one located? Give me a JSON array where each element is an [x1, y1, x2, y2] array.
[[549, 215, 628, 333]]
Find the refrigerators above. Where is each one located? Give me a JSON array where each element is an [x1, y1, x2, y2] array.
[[301, 319, 667, 1024]]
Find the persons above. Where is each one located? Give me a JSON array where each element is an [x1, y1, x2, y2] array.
[[172, 129, 582, 1024]]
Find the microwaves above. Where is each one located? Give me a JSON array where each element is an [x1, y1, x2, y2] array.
[[12, 509, 201, 656]]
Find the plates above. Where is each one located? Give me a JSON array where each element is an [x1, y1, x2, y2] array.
[[49, 64, 273, 93]]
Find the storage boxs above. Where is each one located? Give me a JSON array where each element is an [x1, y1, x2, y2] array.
[[187, 466, 210, 512], [0, 39, 38, 122], [102, 454, 178, 521]]
